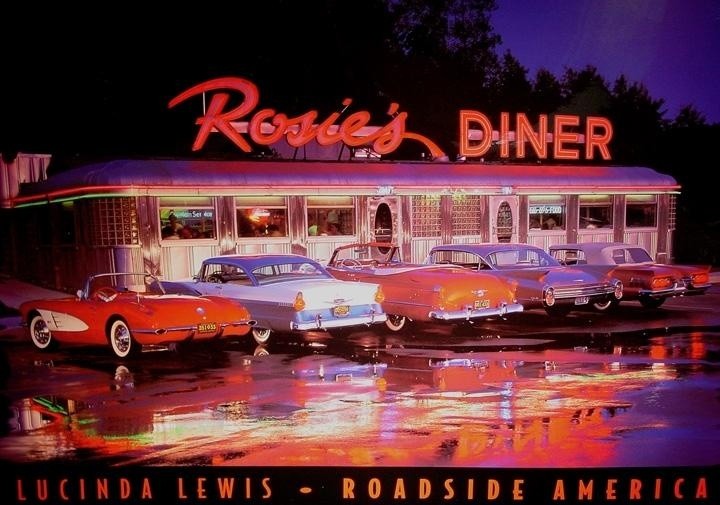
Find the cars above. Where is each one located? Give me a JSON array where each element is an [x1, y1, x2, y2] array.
[[548, 243, 713, 312], [421, 244, 625, 316], [19, 269, 257, 360], [148, 252, 385, 347], [321, 242, 524, 335]]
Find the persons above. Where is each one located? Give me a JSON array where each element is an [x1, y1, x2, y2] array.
[[161, 206, 346, 241], [529, 214, 641, 230]]
